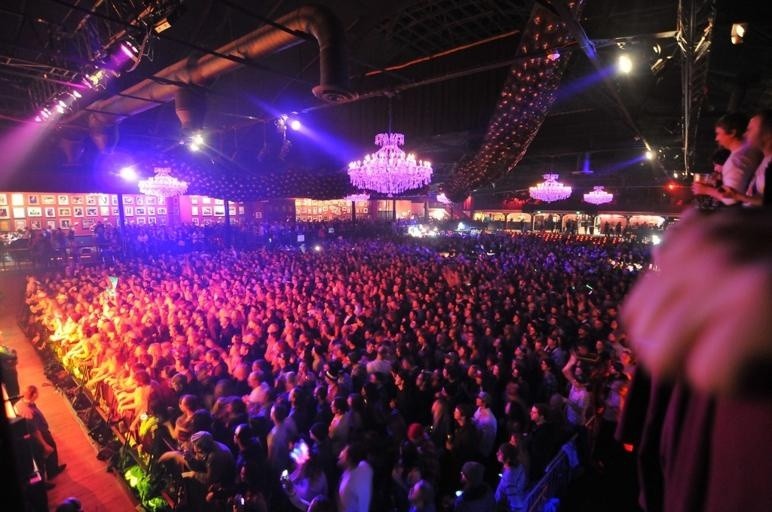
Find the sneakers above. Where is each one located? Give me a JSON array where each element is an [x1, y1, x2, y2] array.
[[47, 463, 66, 480]]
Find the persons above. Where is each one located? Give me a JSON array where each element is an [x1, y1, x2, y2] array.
[[699, 148, 731, 211], [718, 110, 770, 213], [690, 114, 763, 210], [14, 386, 67, 480], [27, 214, 770, 511]]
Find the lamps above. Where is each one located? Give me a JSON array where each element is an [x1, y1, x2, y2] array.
[[28, 35, 147, 124], [529, 171, 614, 205], [346, 104, 433, 200], [139, 167, 190, 201], [598, 22, 751, 78]]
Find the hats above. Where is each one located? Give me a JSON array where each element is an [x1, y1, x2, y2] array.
[[575, 374, 590, 383], [309, 422, 327, 439], [499, 442, 519, 459], [324, 369, 338, 382], [476, 392, 493, 405], [461, 460, 483, 481]]
